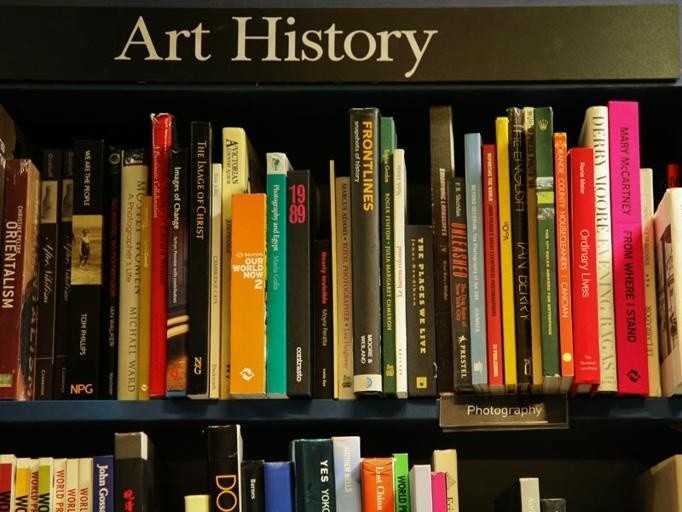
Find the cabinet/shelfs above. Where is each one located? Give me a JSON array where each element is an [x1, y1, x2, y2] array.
[[0, 101, 681, 511]]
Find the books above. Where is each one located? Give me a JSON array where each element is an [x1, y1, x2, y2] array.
[[2, 99, 682, 402], [1, 433, 682, 512]]
